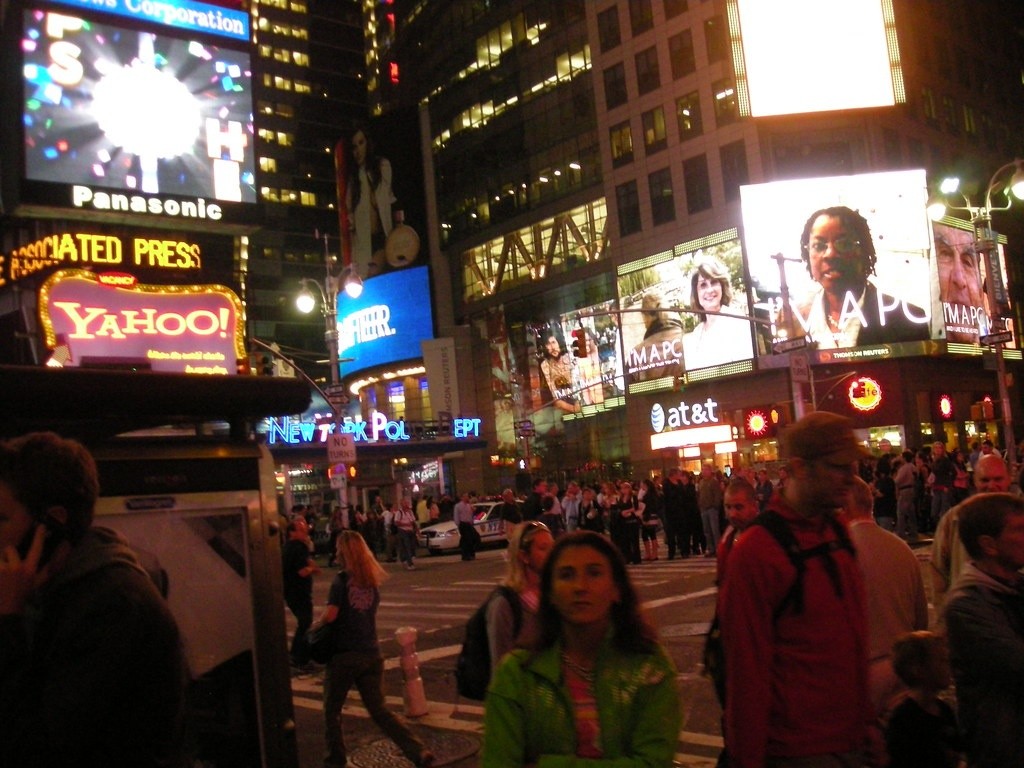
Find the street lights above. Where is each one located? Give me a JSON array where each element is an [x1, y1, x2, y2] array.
[[927, 157, 1024, 488], [294, 260, 365, 529]]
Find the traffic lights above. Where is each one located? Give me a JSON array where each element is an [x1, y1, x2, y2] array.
[[931, 390, 956, 425], [571, 328, 587, 358], [348, 466, 357, 478], [742, 404, 774, 441]]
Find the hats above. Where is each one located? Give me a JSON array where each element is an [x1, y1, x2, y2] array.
[[779, 411, 875, 466]]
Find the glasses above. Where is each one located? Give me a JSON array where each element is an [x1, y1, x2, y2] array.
[[519, 521, 547, 548]]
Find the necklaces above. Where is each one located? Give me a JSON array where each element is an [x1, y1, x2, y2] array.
[[563, 655, 590, 674]]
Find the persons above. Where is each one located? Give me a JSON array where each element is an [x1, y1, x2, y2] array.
[[942, 494, 1023, 768], [716, 437, 1004, 545], [412, 463, 722, 563], [480, 532, 682, 768], [308, 531, 433, 768], [683, 260, 754, 370], [540, 300, 624, 413], [706, 481, 760, 707], [282, 496, 421, 568], [345, 126, 393, 276], [723, 412, 887, 768], [791, 206, 928, 348], [888, 630, 965, 768], [485, 522, 554, 664], [283, 522, 318, 672], [838, 476, 929, 700], [0, 433, 198, 768], [932, 222, 983, 343], [626, 290, 686, 381], [930, 457, 1024, 621]]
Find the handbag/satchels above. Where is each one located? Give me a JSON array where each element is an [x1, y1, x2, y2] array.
[[455, 587, 521, 702], [301, 571, 364, 664], [390, 510, 402, 535]]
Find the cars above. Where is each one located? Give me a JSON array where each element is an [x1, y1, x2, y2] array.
[[414, 495, 529, 554]]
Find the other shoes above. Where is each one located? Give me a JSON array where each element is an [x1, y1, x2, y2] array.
[[323, 756, 347, 768], [401, 561, 415, 571], [290, 649, 310, 666], [625, 548, 715, 565], [417, 751, 432, 768], [386, 558, 397, 562]]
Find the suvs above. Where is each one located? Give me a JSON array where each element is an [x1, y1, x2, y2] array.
[[0, 362, 301, 767]]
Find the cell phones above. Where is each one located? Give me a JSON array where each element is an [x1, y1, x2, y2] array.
[[15, 512, 69, 573]]
